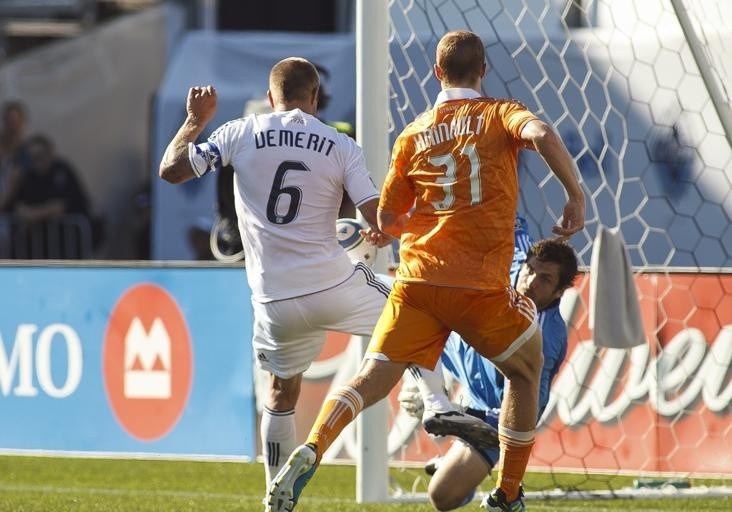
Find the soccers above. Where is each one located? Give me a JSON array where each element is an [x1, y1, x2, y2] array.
[[336, 218, 376, 265]]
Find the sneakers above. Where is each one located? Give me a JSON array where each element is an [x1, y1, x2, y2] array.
[[480, 486, 525, 512], [423, 409, 499, 449], [265, 444, 317, 512]]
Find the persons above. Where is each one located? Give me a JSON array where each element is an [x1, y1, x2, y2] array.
[[3, 133, 102, 258], [158, 54, 496, 510], [263, 33, 584, 511], [401, 211, 577, 510], [1, 98, 33, 207]]
